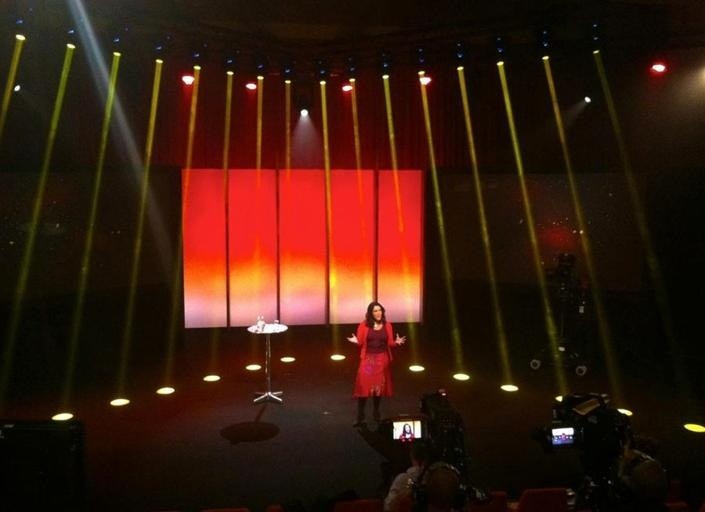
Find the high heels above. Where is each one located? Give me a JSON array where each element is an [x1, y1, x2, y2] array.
[[352, 411, 381, 427]]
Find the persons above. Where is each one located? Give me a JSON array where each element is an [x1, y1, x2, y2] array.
[[559, 434, 569, 443], [384, 444, 434, 511], [423, 466, 459, 512], [400, 424, 415, 439], [346, 302, 407, 426]]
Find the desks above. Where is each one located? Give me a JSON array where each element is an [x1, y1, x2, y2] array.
[[248, 324, 288, 406]]
